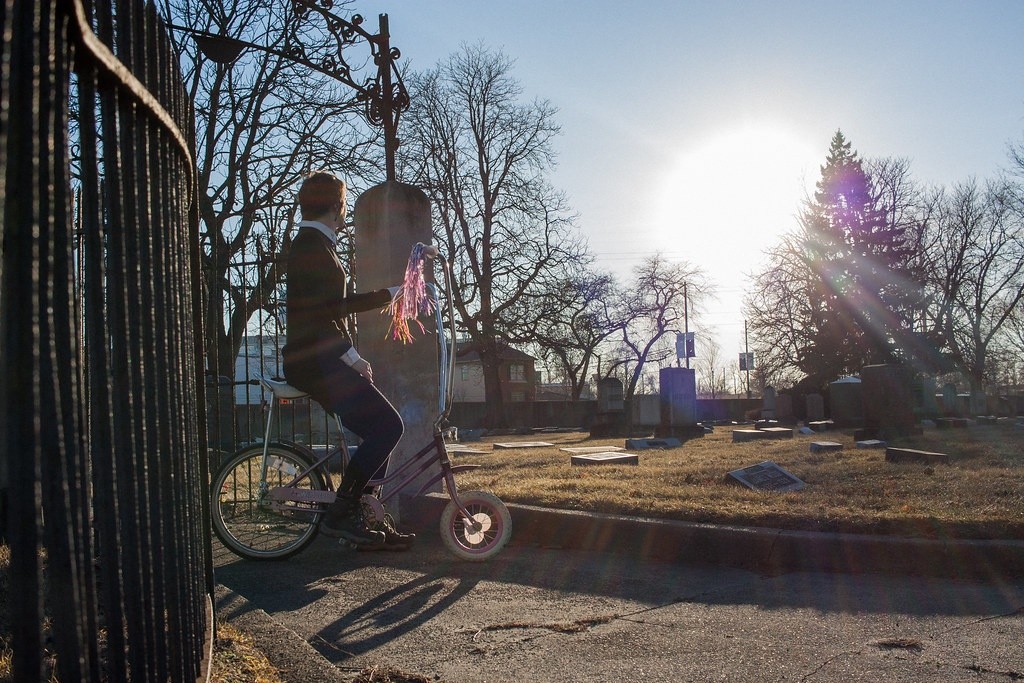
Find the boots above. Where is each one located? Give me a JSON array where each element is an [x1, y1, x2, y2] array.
[[320, 500, 386, 546], [361, 512, 416, 552]]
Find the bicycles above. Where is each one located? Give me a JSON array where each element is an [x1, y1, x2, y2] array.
[[211, 242, 511, 561]]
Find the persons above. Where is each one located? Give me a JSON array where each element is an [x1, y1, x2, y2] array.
[[281, 172, 416, 550]]
